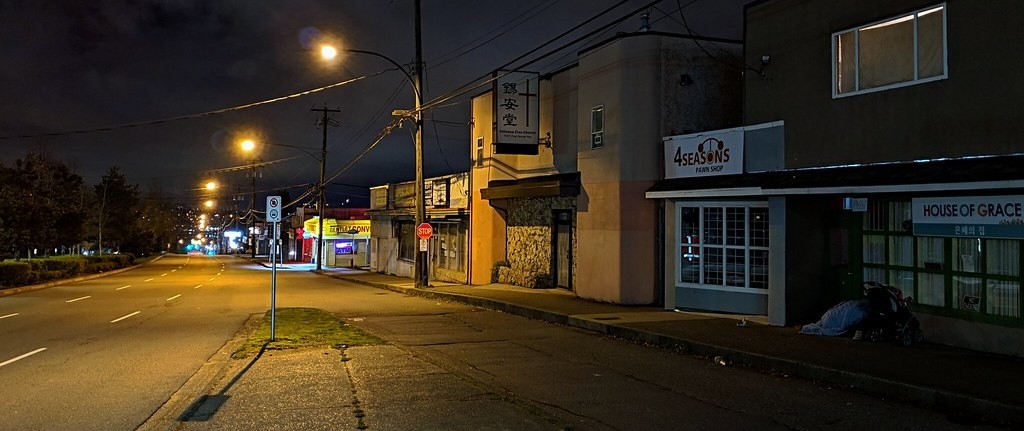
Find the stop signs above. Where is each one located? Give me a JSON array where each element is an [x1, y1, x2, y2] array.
[[416, 223, 433, 240]]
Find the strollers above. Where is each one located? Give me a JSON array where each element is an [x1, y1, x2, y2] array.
[[861, 280, 925, 347]]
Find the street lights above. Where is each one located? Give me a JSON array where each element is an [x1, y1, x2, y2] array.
[[241, 138, 324, 271], [206, 181, 256, 258], [320, 44, 425, 289]]
